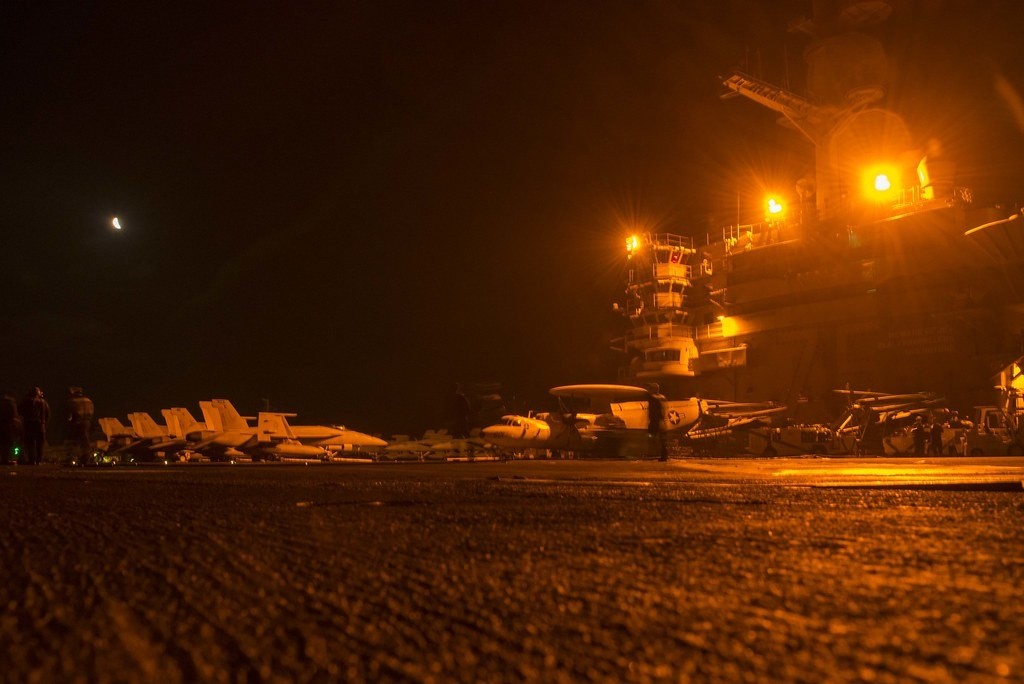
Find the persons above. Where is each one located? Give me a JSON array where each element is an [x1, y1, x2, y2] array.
[[17, 386, 50, 462], [929, 417, 945, 455], [910, 413, 927, 457], [0, 385, 19, 463], [949, 409, 985, 436], [66, 383, 95, 465], [646, 379, 668, 461]]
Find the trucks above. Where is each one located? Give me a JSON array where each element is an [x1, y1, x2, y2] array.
[[882, 406, 1024, 457]]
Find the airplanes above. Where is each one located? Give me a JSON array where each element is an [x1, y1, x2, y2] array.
[[384, 385, 790, 460], [93, 398, 390, 464], [748, 424, 860, 458]]
